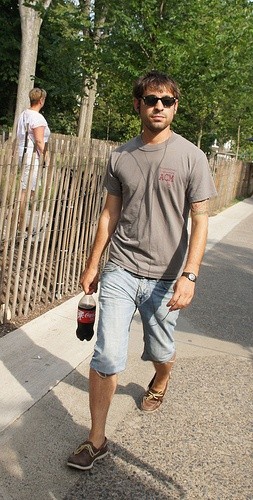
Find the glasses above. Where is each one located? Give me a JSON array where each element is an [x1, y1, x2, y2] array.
[[140, 95, 178, 106]]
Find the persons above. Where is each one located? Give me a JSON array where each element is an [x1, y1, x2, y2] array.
[[15, 88, 52, 239], [66, 71, 219, 470]]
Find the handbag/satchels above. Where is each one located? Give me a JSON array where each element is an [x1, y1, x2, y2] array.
[[43, 161, 45, 168]]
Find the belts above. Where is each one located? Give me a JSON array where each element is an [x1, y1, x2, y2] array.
[[124, 269, 175, 281]]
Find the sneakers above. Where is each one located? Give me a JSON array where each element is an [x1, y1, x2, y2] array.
[[141, 372, 169, 413], [66, 437, 108, 470]]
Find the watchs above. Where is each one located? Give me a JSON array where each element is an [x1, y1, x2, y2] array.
[[182, 272, 197, 282]]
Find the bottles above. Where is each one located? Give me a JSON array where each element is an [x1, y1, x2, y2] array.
[[76, 287, 96, 342]]
